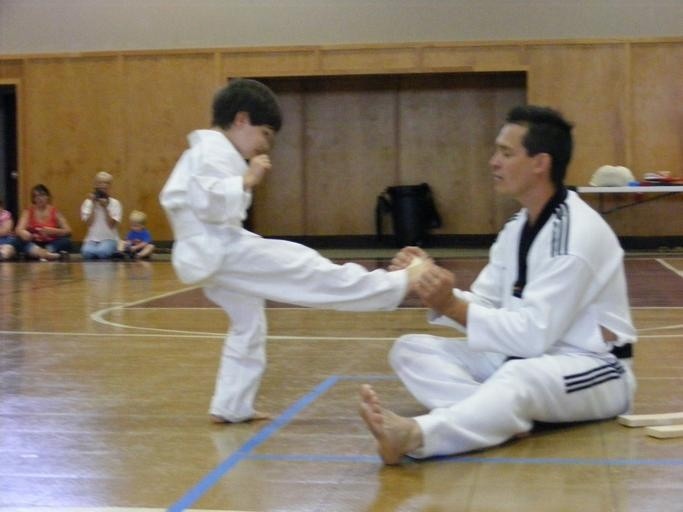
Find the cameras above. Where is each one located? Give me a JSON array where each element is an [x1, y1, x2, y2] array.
[[95, 190, 108, 198]]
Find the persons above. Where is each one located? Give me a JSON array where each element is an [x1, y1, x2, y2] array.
[[15, 183, 71, 262], [160, 78, 436, 423], [123, 210, 155, 260], [77, 172, 123, 258], [354, 104, 639, 464], [0, 191, 20, 261]]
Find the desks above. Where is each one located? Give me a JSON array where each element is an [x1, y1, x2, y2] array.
[[568, 184, 683, 221]]
